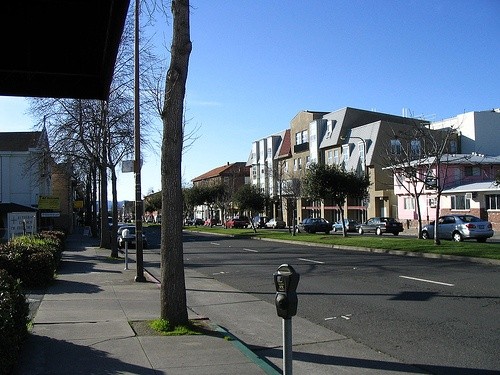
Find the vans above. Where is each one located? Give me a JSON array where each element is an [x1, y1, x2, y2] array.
[[97, 216, 114, 234]]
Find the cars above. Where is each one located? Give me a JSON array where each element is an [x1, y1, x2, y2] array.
[[421, 214, 494, 242], [251, 216, 269, 228], [117, 214, 162, 224], [108, 223, 128, 237], [332, 219, 360, 233], [204, 219, 217, 226], [297, 217, 332, 234], [265, 218, 286, 229], [225, 216, 250, 228], [190, 218, 205, 226], [116, 226, 148, 248], [356, 217, 404, 235]]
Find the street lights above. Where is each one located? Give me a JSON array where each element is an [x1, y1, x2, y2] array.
[[340, 135, 368, 222]]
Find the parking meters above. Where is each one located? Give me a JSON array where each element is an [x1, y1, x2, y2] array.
[[122, 229, 129, 271], [273, 264, 302, 375]]
